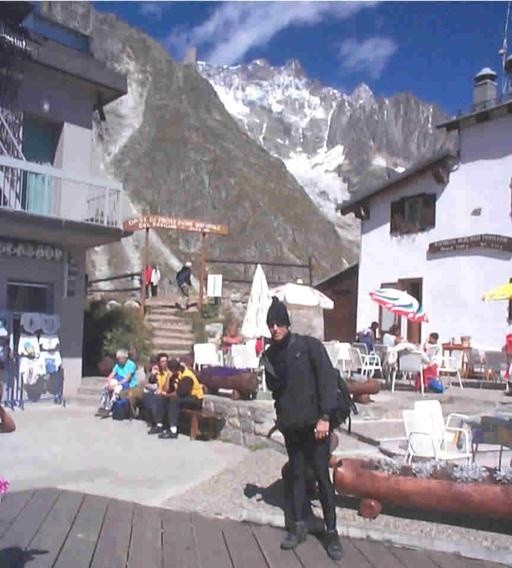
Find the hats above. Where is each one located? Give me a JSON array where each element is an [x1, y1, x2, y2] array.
[[266, 294, 290, 324]]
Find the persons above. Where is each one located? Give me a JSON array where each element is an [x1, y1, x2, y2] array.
[[383, 324, 401, 346], [150, 264, 161, 297], [368, 321, 378, 340], [422, 332, 443, 370], [176, 261, 192, 310], [94, 348, 204, 439], [261, 296, 343, 560], [144, 264, 151, 298]]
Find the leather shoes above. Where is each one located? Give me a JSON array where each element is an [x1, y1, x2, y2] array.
[[159, 429, 178, 439], [148, 424, 164, 435]]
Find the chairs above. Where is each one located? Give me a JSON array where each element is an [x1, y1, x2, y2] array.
[[400, 397, 475, 472], [190, 339, 270, 392], [322, 339, 469, 397]]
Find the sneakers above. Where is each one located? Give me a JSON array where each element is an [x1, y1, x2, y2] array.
[[95, 407, 112, 418], [324, 530, 343, 561], [280, 523, 308, 551]]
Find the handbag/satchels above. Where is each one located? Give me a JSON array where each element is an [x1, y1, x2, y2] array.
[[306, 337, 353, 435]]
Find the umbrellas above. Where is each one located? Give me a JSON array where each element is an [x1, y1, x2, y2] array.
[[269, 283, 335, 310], [240, 264, 272, 339], [369, 288, 429, 324]]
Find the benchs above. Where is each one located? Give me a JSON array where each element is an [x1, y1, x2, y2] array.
[[179, 403, 226, 441]]
